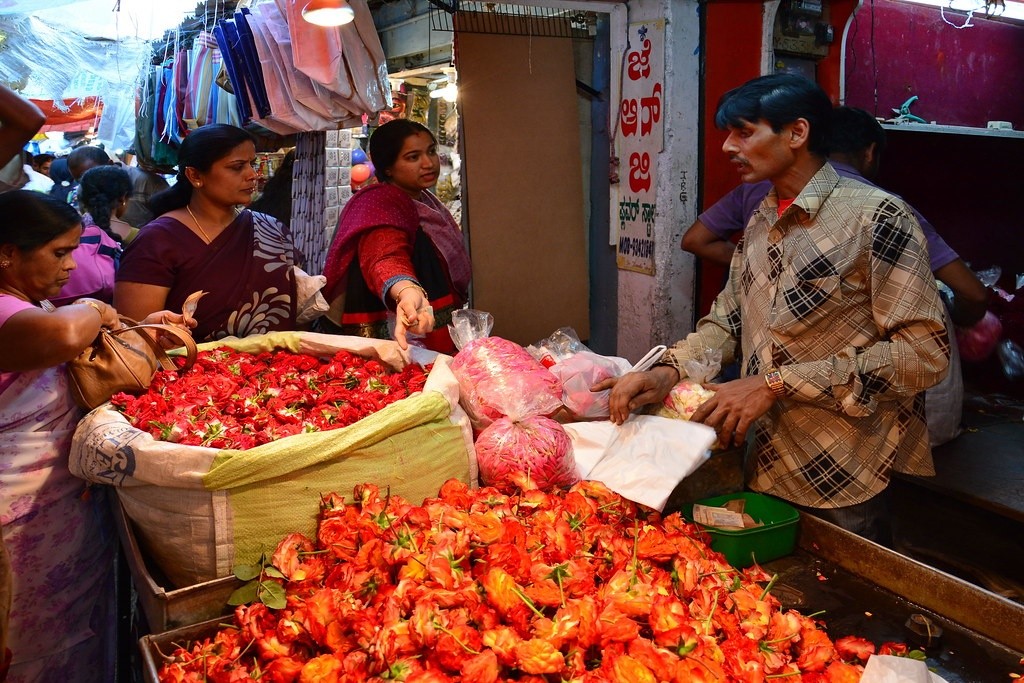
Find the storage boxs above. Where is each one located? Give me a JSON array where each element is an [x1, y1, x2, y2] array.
[[683, 491, 801, 568]]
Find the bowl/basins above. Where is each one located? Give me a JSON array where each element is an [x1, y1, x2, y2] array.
[[681, 492, 800, 569]]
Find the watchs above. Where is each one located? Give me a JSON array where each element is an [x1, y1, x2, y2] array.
[[764, 371, 785, 400]]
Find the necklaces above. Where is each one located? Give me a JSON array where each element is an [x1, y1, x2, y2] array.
[[186, 205, 241, 243]]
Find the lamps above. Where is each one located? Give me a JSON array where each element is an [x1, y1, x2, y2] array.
[[302, 0, 355, 27]]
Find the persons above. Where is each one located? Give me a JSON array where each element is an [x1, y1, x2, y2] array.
[[589, 75, 952, 544], [114, 123, 297, 343], [24, 145, 169, 304], [0, 189, 197, 683], [0, 83, 46, 170], [321, 119, 473, 356], [679, 104, 1008, 306]]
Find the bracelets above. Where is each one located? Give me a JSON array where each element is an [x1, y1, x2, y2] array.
[[78, 301, 103, 320], [395, 285, 428, 305]]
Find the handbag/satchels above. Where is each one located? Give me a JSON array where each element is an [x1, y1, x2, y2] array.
[[63, 316, 197, 409], [133, 0, 395, 173]]
